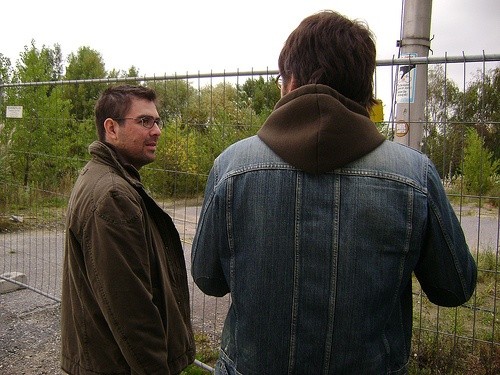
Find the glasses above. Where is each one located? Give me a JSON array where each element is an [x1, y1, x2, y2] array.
[[113, 117, 164, 130], [275, 70, 292, 89]]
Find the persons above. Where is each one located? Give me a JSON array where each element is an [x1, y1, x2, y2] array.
[[59, 84, 197, 375], [190, 11, 476, 375]]
